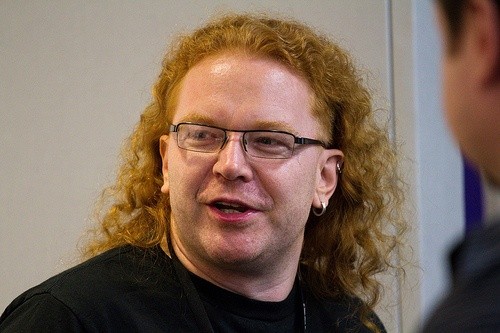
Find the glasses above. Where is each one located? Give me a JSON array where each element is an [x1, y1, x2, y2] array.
[[170, 123, 332, 159]]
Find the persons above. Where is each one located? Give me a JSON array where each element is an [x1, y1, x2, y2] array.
[[0, 12, 408, 333], [420, 0, 500, 333]]
